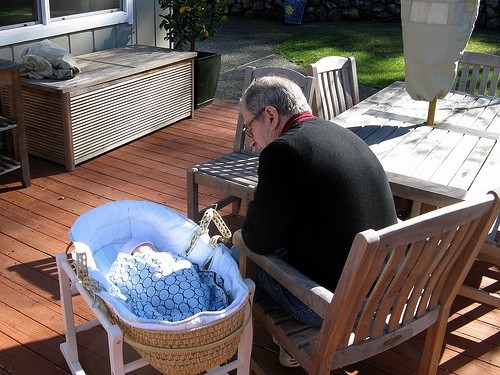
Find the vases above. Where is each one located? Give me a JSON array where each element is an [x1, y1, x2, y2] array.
[[282, 0, 306, 25]]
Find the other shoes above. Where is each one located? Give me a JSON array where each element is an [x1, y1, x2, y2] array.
[[272, 334, 301, 367]]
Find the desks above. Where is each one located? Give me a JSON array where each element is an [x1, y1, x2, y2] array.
[[326, 81, 500, 208]]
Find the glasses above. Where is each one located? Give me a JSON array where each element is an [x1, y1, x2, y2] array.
[[242, 107, 266, 138]]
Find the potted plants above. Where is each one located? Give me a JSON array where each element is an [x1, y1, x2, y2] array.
[[160, 0, 228, 109]]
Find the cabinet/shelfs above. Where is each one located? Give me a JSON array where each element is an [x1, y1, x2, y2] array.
[[0, 56, 31, 188]]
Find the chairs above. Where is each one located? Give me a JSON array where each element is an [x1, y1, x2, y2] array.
[[308, 55, 360, 121], [452, 51, 500, 98], [187, 65, 316, 224], [229, 189, 500, 374]]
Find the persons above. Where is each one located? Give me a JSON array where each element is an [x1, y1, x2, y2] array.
[[230, 77, 397, 369], [120, 238, 153, 255]]
